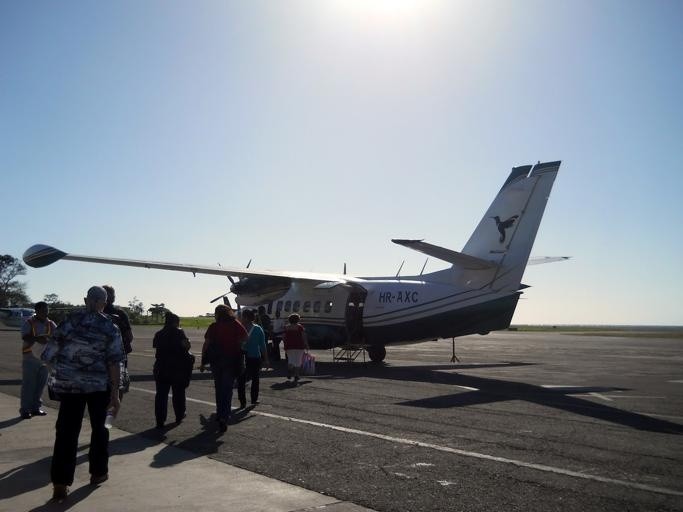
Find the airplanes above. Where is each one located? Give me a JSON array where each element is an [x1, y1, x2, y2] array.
[[22, 159, 575, 364], [0, 298, 85, 330]]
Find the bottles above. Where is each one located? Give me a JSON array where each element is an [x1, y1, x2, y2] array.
[[104, 407, 115, 429]]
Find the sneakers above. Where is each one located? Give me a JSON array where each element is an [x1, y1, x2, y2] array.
[[20, 411, 47, 419], [90, 475, 107, 484]]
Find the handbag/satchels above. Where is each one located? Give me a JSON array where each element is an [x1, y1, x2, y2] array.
[[302, 352, 314, 372]]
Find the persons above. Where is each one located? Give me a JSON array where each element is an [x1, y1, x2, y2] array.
[[99, 285, 134, 403], [258, 306, 273, 346], [152, 312, 191, 427], [197, 305, 249, 432], [19, 302, 57, 419], [283, 314, 309, 380], [41, 286, 130, 498], [234, 310, 267, 409]]
